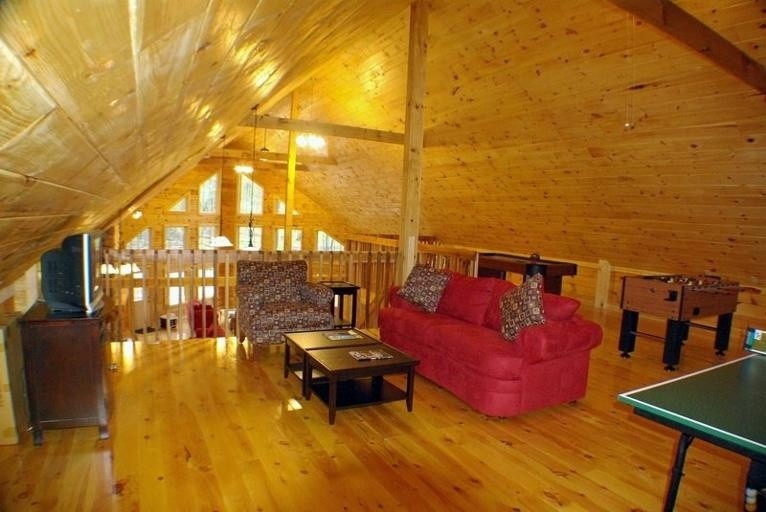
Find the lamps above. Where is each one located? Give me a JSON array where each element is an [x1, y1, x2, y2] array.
[[260, 114, 269, 151], [242, 106, 262, 249], [209, 136, 232, 247], [297, 76, 326, 147]]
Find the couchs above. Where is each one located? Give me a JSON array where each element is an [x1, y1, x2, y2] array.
[[186, 300, 225, 337], [381, 268, 601, 419]]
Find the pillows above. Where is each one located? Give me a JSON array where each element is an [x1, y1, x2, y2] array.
[[398, 264, 451, 313], [499, 272, 547, 341]]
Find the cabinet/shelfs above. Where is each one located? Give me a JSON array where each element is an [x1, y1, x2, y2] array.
[[16, 299, 117, 446]]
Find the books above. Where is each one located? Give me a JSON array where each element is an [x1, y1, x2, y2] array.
[[743, 327, 766, 355], [322, 329, 364, 340], [348, 348, 395, 361]]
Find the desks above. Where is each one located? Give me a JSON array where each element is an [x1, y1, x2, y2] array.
[[478, 251, 577, 295], [321, 280, 360, 329], [618, 326, 766, 510]]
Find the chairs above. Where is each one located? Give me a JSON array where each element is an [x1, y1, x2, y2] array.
[[238, 262, 332, 346]]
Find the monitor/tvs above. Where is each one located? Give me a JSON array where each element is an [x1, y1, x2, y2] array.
[[41, 229, 105, 316]]
